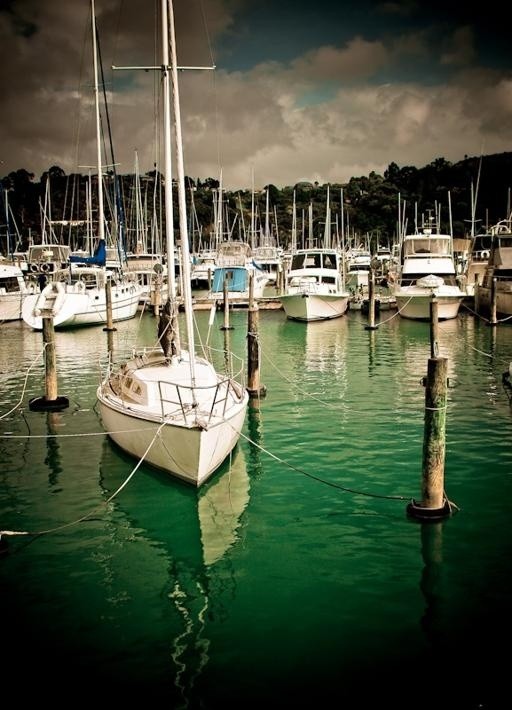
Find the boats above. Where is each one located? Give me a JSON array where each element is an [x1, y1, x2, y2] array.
[[92, 429, 253, 576], [90, 1, 253, 490]]
